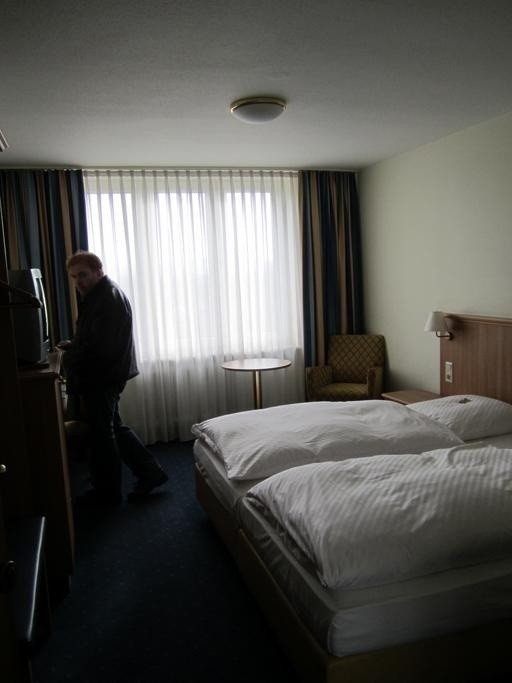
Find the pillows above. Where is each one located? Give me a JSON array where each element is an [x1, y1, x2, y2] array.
[[405, 395, 511, 440]]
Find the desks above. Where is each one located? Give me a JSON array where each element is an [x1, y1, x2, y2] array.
[[221, 358, 293, 409], [0, 516, 54, 682]]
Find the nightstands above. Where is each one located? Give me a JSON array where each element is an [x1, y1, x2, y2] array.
[[382, 388, 439, 404]]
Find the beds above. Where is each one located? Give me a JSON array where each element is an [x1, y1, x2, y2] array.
[[188, 396, 512, 548], [229, 434, 511, 683]]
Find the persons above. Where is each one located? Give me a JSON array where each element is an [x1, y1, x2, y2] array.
[[57, 251, 169, 513]]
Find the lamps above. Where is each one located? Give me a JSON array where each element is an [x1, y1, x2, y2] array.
[[230, 95, 288, 123], [426, 311, 453, 341]]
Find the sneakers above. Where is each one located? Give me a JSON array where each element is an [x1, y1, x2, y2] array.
[[74, 490, 122, 510], [139, 472, 168, 496]]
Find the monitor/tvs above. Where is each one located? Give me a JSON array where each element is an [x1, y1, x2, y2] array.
[[11, 268, 52, 363]]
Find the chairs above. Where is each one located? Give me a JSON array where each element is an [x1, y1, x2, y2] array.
[[303, 332, 386, 401]]
